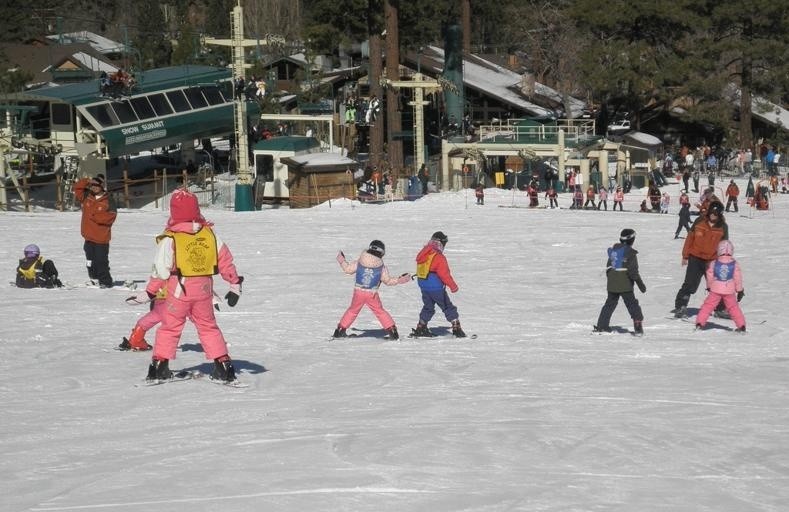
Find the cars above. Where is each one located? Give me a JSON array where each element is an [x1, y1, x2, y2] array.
[[608, 120, 631, 134]]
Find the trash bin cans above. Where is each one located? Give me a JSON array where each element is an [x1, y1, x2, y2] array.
[[407, 175, 419, 195], [592, 172, 602, 193], [555, 181, 563, 192], [235, 183, 255, 211]]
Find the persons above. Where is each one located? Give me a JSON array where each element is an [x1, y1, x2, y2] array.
[[410, 231, 467, 337], [418, 164, 428, 195], [345, 95, 393, 195], [333, 240, 412, 340], [476, 176, 485, 205], [425, 113, 475, 156]]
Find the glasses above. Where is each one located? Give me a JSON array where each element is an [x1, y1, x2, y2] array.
[[707, 207, 723, 214]]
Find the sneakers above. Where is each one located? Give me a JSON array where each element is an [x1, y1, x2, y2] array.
[[418, 321, 433, 334], [100, 281, 112, 287], [452, 321, 466, 336], [334, 326, 348, 338], [675, 305, 747, 331], [388, 326, 398, 340], [86, 278, 99, 286]]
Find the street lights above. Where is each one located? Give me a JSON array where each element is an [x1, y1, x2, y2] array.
[[416, 46, 424, 73]]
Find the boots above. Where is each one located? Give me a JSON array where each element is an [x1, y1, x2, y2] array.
[[212, 355, 235, 381], [598, 319, 611, 330], [634, 319, 643, 334], [128, 322, 153, 349], [147, 358, 174, 378]]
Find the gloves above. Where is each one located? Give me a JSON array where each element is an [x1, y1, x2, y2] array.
[[707, 288, 710, 292], [736, 289, 745, 303], [224, 276, 244, 306], [398, 272, 411, 284], [336, 250, 345, 265], [147, 291, 155, 310]]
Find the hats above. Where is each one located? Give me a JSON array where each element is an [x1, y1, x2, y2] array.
[[717, 239, 734, 256], [367, 239, 385, 256], [24, 243, 40, 259], [708, 201, 725, 216], [90, 173, 105, 190], [170, 188, 201, 222], [620, 229, 637, 242]]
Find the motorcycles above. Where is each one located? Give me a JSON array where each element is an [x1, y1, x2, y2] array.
[[623, 180, 631, 193]]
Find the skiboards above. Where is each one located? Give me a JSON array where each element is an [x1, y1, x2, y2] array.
[[135, 371, 248, 388], [67, 281, 137, 290]]
